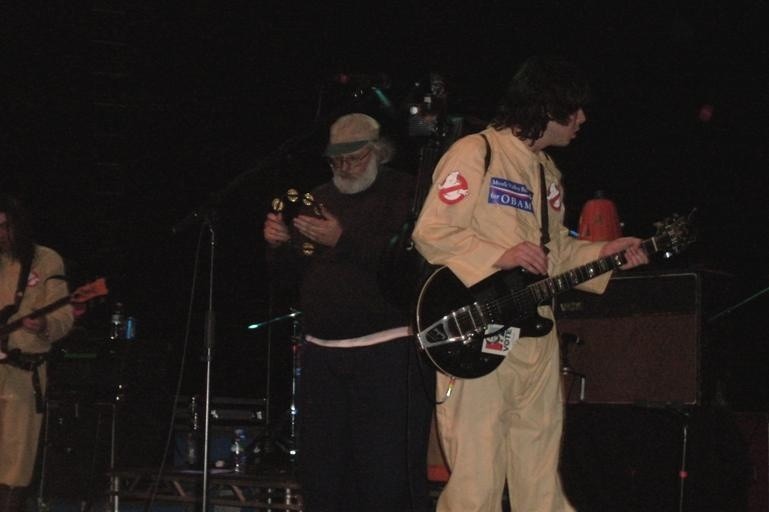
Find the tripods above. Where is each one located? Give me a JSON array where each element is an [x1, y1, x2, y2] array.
[[236, 253, 293, 462]]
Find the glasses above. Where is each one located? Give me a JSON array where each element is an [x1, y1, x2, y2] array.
[[328, 149, 373, 167]]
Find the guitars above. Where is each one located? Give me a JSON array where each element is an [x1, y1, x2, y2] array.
[[1, 276, 111, 349], [413, 222, 696, 377]]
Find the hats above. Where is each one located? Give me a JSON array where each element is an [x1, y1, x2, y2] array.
[[324, 113, 379, 157]]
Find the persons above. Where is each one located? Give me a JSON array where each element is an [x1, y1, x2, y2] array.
[[1, 193, 75, 511], [411, 51, 650, 512], [263, 113, 437, 512]]
